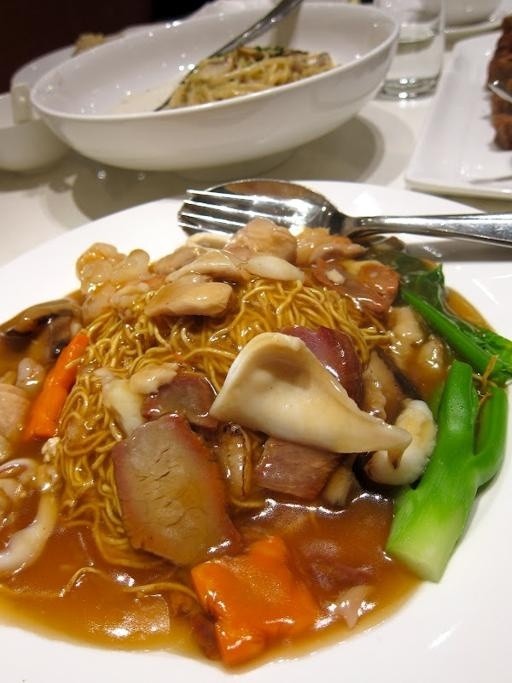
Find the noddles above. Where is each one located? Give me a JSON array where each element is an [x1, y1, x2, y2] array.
[[51, 276, 394, 606]]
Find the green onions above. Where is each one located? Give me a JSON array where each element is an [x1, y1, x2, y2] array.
[[385, 359, 508, 583]]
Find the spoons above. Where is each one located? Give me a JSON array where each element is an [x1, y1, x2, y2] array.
[[177, 178, 512, 243]]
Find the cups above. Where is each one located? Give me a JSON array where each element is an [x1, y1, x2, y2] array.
[[375, 0, 446, 99]]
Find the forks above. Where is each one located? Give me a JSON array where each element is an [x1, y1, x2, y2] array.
[[178, 184, 512, 257]]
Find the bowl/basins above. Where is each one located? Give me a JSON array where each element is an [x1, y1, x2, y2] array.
[[28, 1, 402, 184], [1, 90, 68, 179], [419, 0, 499, 24]]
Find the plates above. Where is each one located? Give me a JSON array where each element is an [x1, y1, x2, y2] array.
[[411, 30, 510, 202], [10, 33, 122, 90], [1, 176, 507, 678], [442, 6, 505, 42]]
